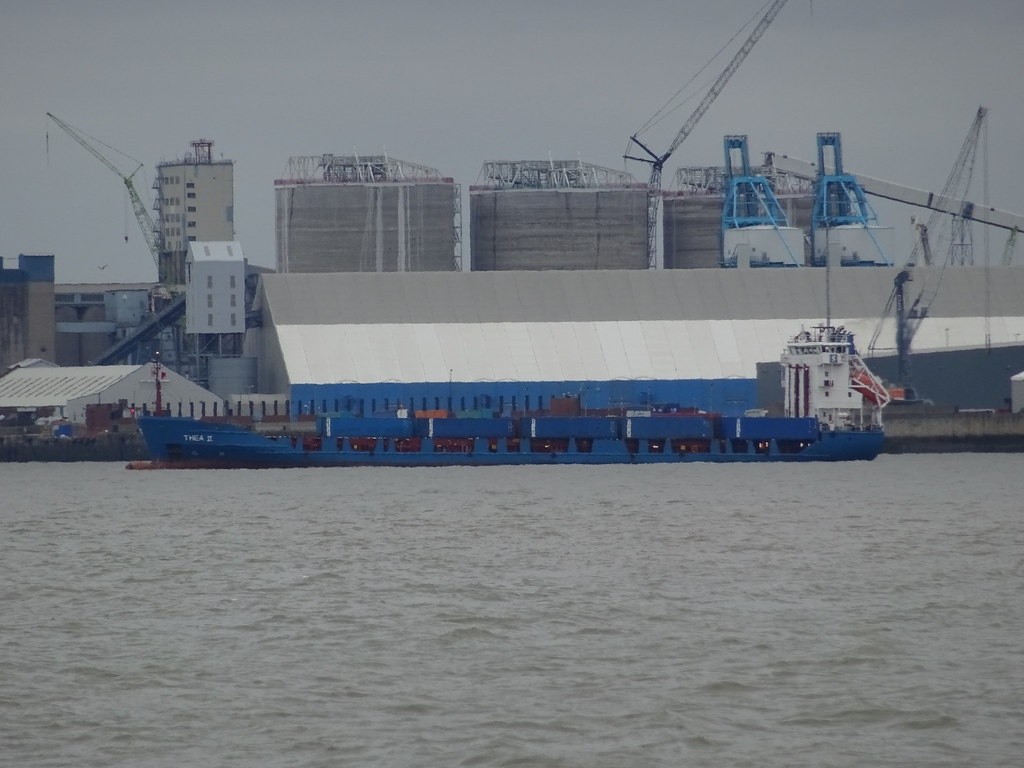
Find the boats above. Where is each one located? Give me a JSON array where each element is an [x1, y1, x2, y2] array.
[[133, 315, 892, 469]]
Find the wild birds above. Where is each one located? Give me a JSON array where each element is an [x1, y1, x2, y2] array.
[[98, 264, 108, 270]]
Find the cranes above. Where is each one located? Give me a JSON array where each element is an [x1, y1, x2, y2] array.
[[45, 112, 186, 302], [622, 0, 788, 270]]
